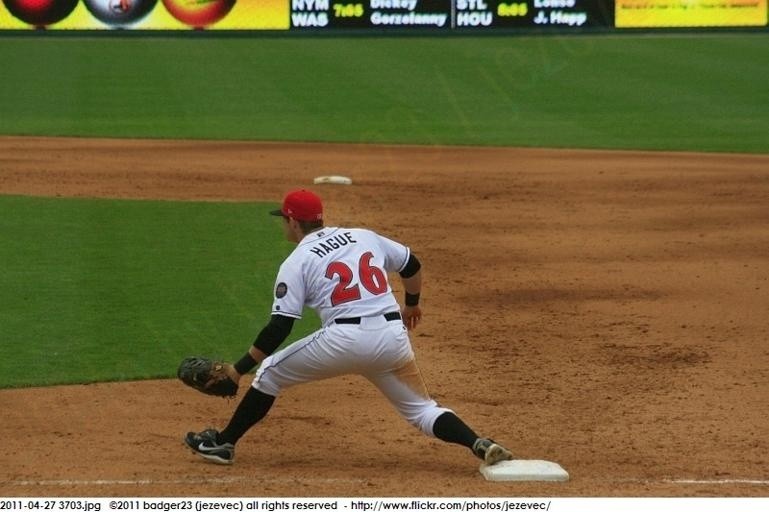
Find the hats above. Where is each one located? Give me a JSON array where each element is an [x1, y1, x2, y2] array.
[[267, 188, 323, 222]]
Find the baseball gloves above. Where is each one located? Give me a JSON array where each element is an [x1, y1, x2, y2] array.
[[178, 357, 238, 396]]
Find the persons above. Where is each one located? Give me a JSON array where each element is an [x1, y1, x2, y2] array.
[[176, 189, 514, 465]]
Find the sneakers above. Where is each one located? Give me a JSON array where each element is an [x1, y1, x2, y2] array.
[[184, 429, 239, 467], [472, 435, 514, 466]]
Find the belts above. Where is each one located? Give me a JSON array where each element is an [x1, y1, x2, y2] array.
[[332, 312, 404, 325]]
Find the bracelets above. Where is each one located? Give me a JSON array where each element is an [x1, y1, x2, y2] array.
[[404, 290, 421, 307], [232, 349, 260, 377]]
